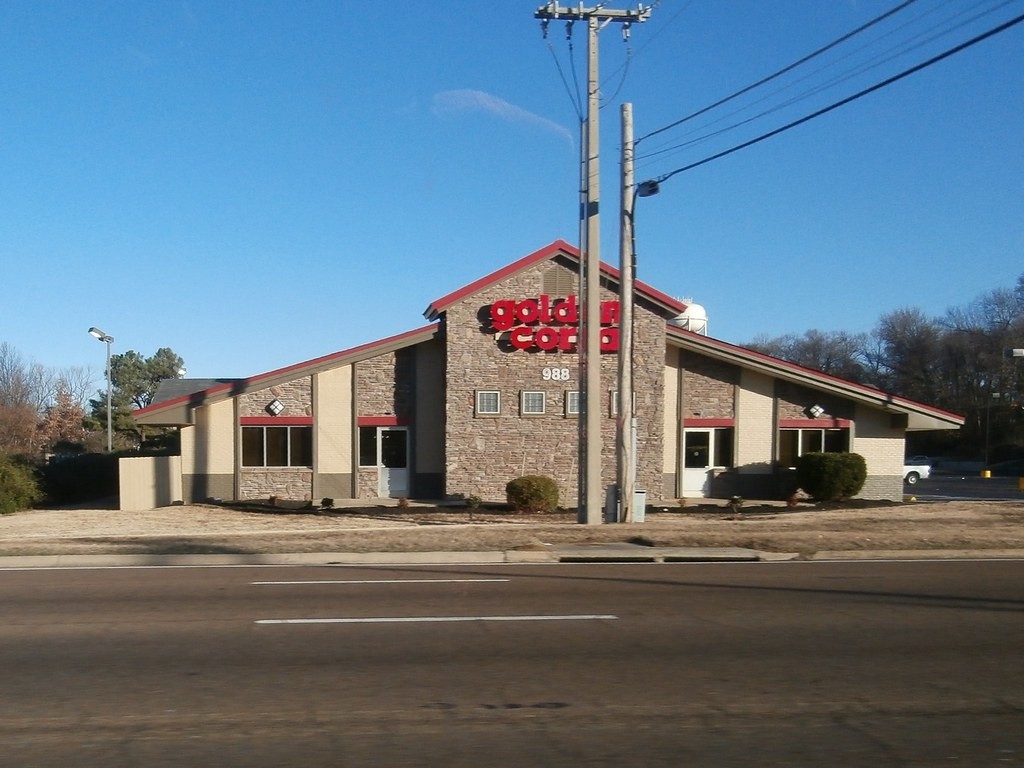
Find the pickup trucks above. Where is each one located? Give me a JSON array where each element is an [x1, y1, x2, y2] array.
[[903, 465, 931, 485]]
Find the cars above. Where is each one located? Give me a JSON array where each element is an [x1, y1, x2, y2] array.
[[911, 456, 933, 467]]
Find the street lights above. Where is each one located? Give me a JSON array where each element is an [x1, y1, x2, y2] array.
[[983, 392, 1001, 469], [89, 328, 115, 452], [621, 182, 660, 520]]
